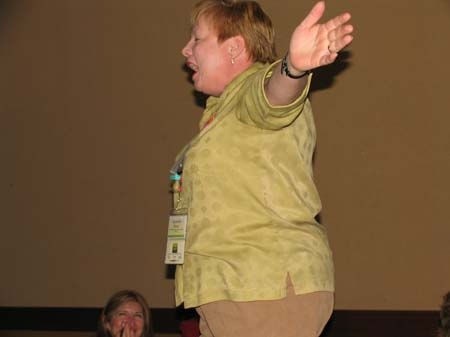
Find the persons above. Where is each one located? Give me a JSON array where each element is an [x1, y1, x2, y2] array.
[[158, 2, 355, 335], [102, 291, 150, 337]]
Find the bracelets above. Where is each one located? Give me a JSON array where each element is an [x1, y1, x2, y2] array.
[[280, 52, 311, 79]]
